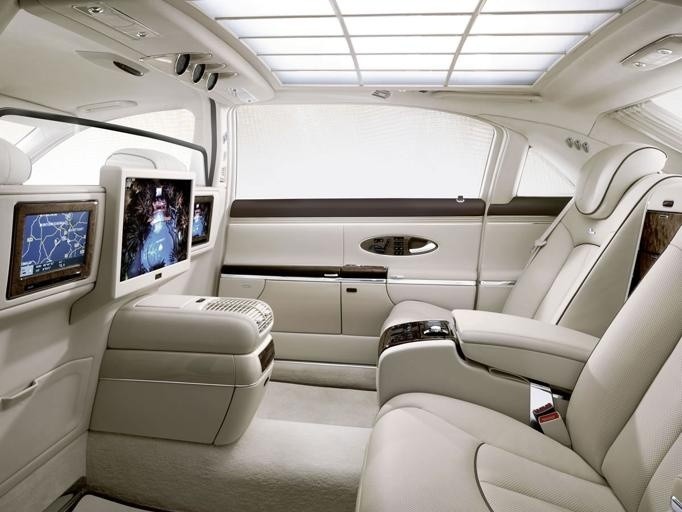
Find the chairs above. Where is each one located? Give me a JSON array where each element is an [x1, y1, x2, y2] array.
[[372, 141, 670, 340], [357, 227, 680, 511]]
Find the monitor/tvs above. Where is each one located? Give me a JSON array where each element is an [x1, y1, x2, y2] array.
[[114, 169, 196, 300], [191, 196, 214, 248], [6, 200, 98, 300]]
